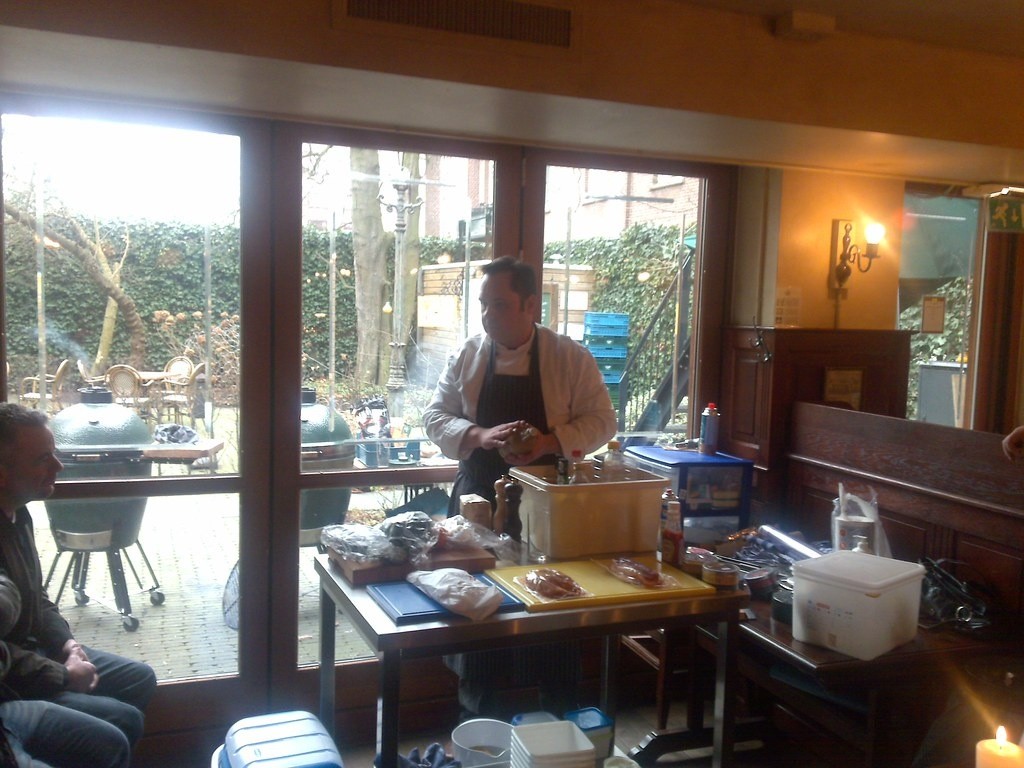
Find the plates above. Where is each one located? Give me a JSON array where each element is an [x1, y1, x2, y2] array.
[[509, 719, 596, 768]]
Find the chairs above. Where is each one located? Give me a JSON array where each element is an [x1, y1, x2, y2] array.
[[161, 364, 208, 429], [104, 365, 155, 430], [153, 356, 194, 425], [20, 359, 70, 414], [76, 359, 107, 387]]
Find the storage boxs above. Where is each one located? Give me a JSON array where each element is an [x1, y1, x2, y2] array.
[[792, 548, 927, 661], [584, 311, 629, 337], [595, 358, 626, 383], [509, 466, 671, 560], [582, 334, 627, 358], [605, 383, 619, 410], [625, 445, 755, 534]]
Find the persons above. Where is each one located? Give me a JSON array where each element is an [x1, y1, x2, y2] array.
[[1001, 425, 1024, 463], [0, 570, 148, 768], [1, 400, 158, 756], [421, 253, 618, 552]]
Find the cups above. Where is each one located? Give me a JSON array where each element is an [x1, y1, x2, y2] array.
[[834, 515, 875, 552], [527, 510, 551, 563]]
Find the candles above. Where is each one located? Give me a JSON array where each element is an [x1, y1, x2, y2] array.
[[974, 726, 1024, 768]]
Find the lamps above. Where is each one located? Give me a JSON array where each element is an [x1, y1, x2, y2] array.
[[835, 216, 887, 287]]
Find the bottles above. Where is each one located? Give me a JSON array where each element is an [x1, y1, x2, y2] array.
[[602, 440, 625, 481], [567, 448, 590, 483], [698, 402, 721, 455], [662, 500, 684, 568], [658, 487, 680, 555]]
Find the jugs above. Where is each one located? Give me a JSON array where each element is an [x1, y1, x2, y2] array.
[[451, 718, 516, 768]]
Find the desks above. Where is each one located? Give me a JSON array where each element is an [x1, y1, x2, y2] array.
[[106, 371, 179, 425], [44, 439, 225, 633], [621, 598, 1008, 754], [312, 552, 755, 768]]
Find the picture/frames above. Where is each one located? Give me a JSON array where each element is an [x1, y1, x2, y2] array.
[[821, 366, 865, 411]]
